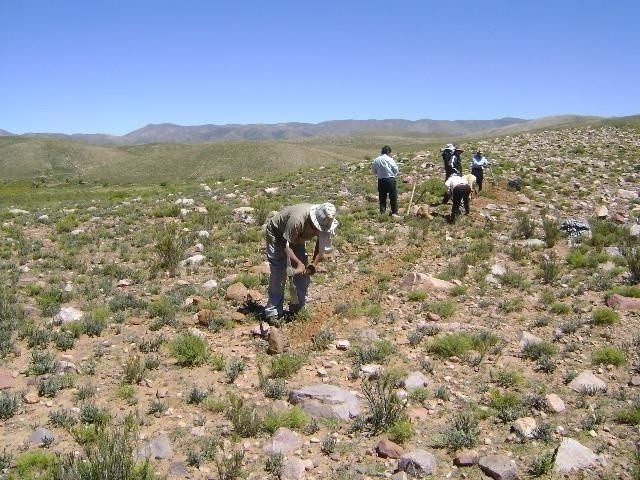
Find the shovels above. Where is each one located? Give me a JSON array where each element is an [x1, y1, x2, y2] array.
[[404, 182, 417, 222], [489, 167, 498, 188]]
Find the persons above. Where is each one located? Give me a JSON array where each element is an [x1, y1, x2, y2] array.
[[472, 148, 491, 192], [261, 203, 339, 326], [443, 145, 466, 206], [372, 146, 403, 219], [441, 142, 456, 204], [444, 171, 472, 224], [459, 172, 478, 213]]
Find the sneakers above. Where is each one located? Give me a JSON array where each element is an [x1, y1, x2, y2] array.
[[269, 315, 286, 326]]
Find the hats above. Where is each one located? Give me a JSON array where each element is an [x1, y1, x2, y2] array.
[[444, 143, 465, 154], [310, 202, 340, 234]]
